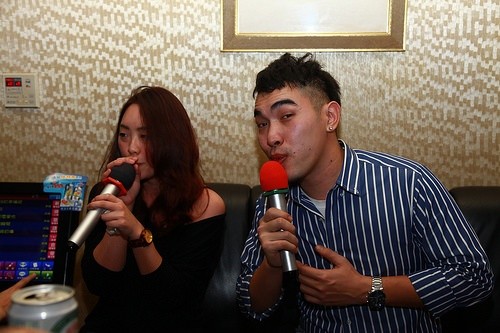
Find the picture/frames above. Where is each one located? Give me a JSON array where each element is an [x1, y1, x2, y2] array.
[[220, 0, 408, 53]]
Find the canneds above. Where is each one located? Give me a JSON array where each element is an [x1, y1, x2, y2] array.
[[6, 284, 80, 333]]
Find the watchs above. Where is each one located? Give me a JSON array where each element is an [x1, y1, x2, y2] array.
[[364, 274, 389, 312], [127, 227, 154, 248]]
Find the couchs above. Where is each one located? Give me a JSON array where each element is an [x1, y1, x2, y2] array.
[[207, 182, 500, 333]]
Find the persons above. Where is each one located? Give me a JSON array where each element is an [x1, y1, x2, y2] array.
[[77, 85, 227, 333], [234, 51, 495, 333]]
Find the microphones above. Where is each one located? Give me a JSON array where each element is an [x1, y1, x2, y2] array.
[[68, 163, 136, 248], [260, 160, 298, 272]]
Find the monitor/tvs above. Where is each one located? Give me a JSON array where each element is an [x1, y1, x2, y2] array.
[[0, 181, 80, 295]]
